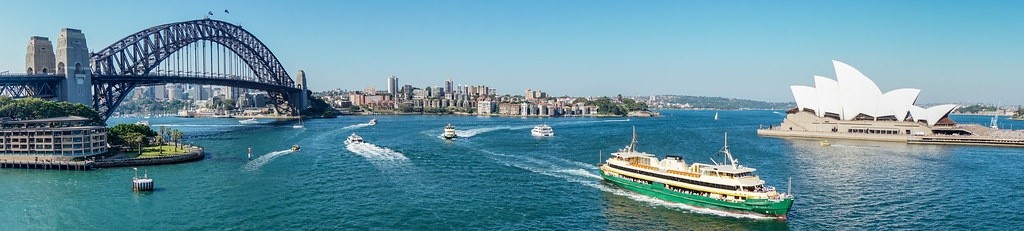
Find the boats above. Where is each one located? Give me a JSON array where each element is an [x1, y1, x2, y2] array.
[[594, 125, 795, 221], [132, 168, 155, 193], [289, 118, 555, 159]]
